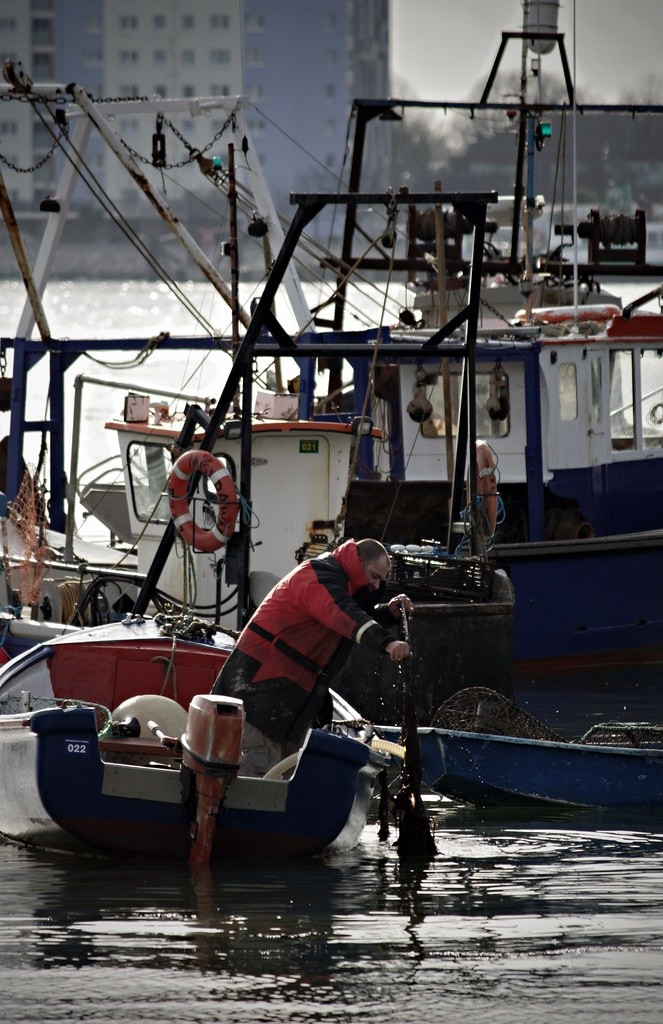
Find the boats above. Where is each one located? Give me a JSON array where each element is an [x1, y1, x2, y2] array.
[[0, 3, 663, 866]]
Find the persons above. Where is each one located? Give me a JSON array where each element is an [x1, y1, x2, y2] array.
[[209, 539, 414, 778]]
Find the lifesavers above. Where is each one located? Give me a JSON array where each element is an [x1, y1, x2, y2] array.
[[461, 435, 501, 546], [513, 301, 625, 328], [165, 446, 244, 555]]
